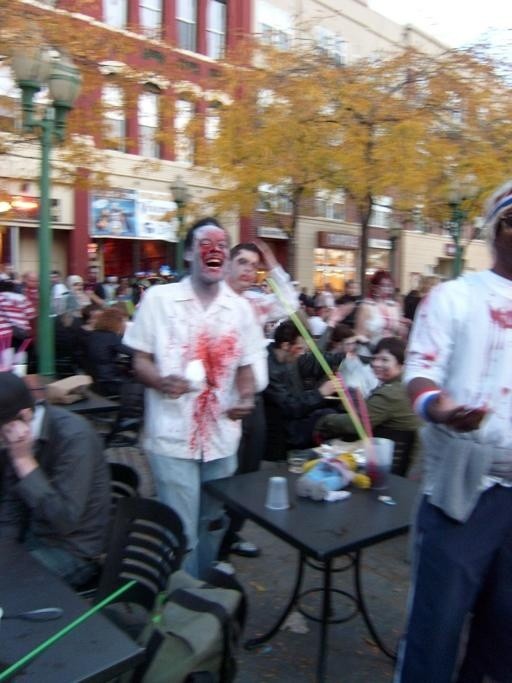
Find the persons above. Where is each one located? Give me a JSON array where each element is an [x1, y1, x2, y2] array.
[[97, 202, 133, 235], [2, 371, 113, 593], [1, 243, 446, 575], [120, 215, 261, 580], [395, 180, 511, 682]]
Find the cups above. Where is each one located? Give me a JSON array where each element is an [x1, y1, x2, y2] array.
[[264, 477, 291, 509], [363, 436, 395, 489], [289, 449, 308, 472]]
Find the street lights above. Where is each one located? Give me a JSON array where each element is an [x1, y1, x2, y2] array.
[[9, 44, 84, 377], [388, 221, 404, 281], [447, 189, 471, 278], [171, 179, 194, 277]]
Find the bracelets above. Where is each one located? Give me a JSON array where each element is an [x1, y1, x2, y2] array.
[[409, 388, 445, 424]]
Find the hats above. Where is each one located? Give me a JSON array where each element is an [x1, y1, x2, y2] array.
[[490, 183, 512, 219]]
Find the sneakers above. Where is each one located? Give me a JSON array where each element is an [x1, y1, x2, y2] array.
[[213, 561, 236, 576], [228, 541, 259, 557]]
